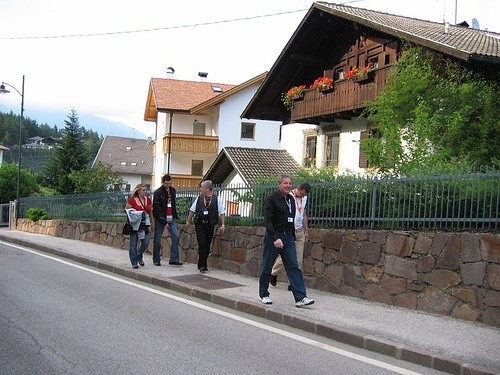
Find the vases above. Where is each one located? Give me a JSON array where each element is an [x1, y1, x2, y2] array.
[[292, 93, 303, 99], [318, 85, 333, 93], [352, 74, 368, 81]]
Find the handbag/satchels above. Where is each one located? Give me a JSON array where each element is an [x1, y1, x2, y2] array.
[[123, 216, 133, 235]]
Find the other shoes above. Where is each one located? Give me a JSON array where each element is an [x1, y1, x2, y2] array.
[[153, 260, 160, 266], [137, 256, 144, 266], [131, 260, 138, 269], [169, 261, 183, 266], [270, 274, 277, 286], [287, 285, 292, 291], [200, 267, 209, 272]]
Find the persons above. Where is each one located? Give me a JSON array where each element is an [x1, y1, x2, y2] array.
[[125, 184, 152, 269], [152, 174, 184, 266], [186, 180, 225, 273], [258, 174, 315, 306], [270, 182, 311, 291]]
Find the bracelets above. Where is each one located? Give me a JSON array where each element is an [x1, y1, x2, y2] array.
[[222, 225, 225, 226]]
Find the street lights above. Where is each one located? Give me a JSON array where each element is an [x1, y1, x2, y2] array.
[[0, 74, 24, 231]]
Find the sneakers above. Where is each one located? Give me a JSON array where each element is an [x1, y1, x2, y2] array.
[[296, 297, 315, 307], [259, 297, 272, 304]]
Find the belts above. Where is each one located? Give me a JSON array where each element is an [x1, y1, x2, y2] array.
[[198, 220, 209, 223]]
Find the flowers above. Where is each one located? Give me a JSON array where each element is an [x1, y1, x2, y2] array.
[[344, 62, 372, 79], [281, 84, 308, 111], [310, 77, 333, 90]]
